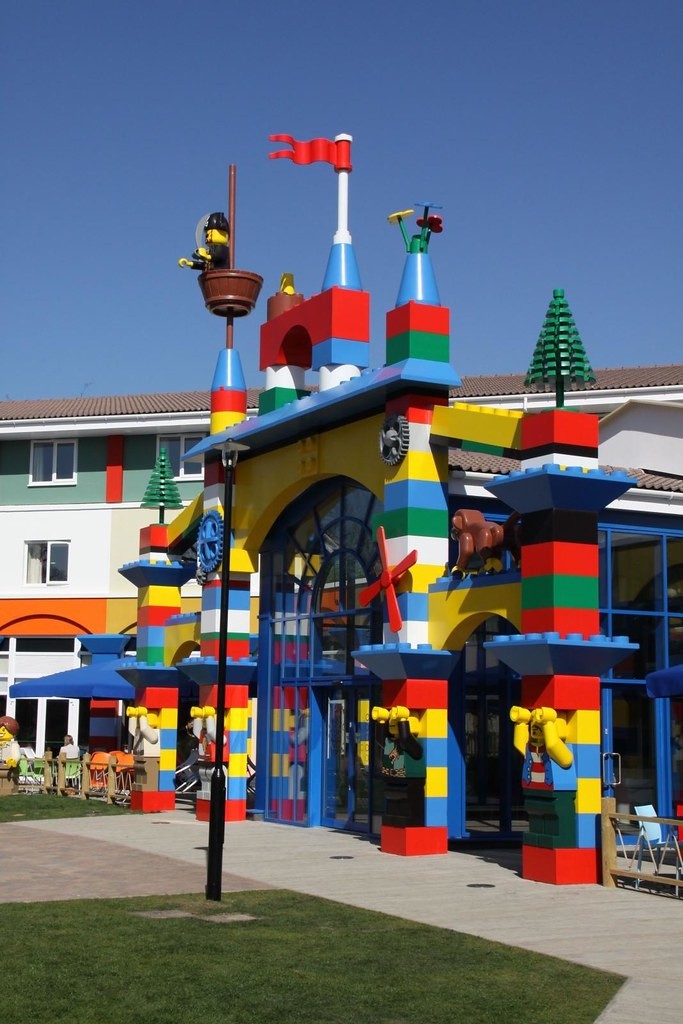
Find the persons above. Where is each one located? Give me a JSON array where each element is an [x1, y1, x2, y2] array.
[[178, 718, 197, 761], [59, 735, 80, 758]]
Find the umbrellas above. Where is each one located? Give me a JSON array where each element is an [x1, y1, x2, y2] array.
[[10, 656, 198, 754]]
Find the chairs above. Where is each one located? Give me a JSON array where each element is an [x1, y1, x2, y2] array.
[[175, 751, 200, 793], [634, 805, 683, 892], [247, 755, 256, 794], [675, 804, 683, 897], [19, 751, 135, 807]]
